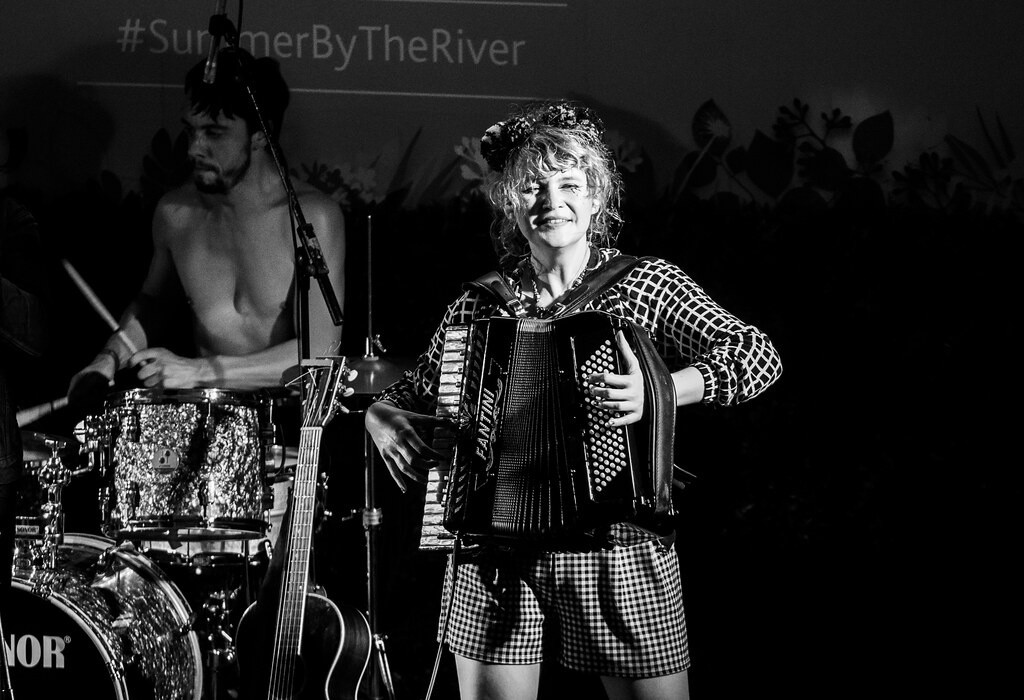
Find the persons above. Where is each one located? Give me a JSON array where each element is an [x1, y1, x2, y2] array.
[[71, 47, 348, 398], [366, 100, 783, 700]]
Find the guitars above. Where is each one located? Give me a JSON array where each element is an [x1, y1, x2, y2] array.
[[232, 355, 376, 700]]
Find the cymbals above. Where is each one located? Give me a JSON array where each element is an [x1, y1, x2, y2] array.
[[18, 430, 71, 463], [279, 352, 422, 397]]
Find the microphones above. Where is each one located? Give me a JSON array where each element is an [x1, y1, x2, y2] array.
[[202, 1, 227, 85]]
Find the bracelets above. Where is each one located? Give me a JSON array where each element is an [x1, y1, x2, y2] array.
[[101, 349, 120, 373]]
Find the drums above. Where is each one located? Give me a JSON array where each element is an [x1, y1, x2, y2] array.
[[0, 529, 203, 700], [143, 548, 261, 628], [97, 384, 273, 543]]
